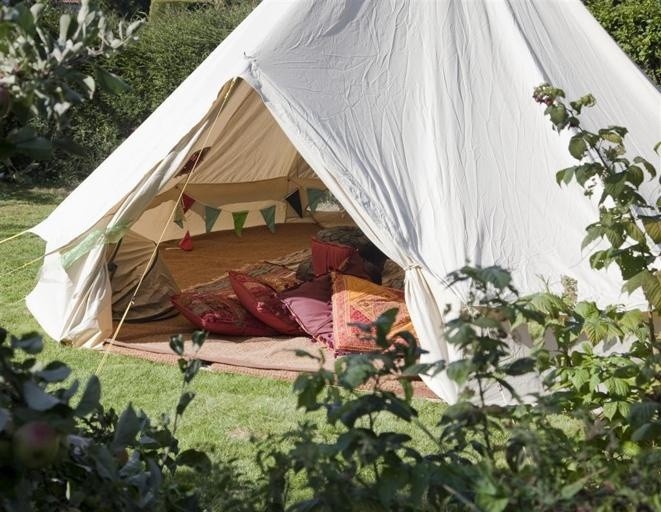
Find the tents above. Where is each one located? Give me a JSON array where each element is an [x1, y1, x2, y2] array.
[[24, 1, 661, 409]]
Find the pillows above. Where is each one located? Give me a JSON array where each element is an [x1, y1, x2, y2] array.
[[168, 251, 420, 358]]
